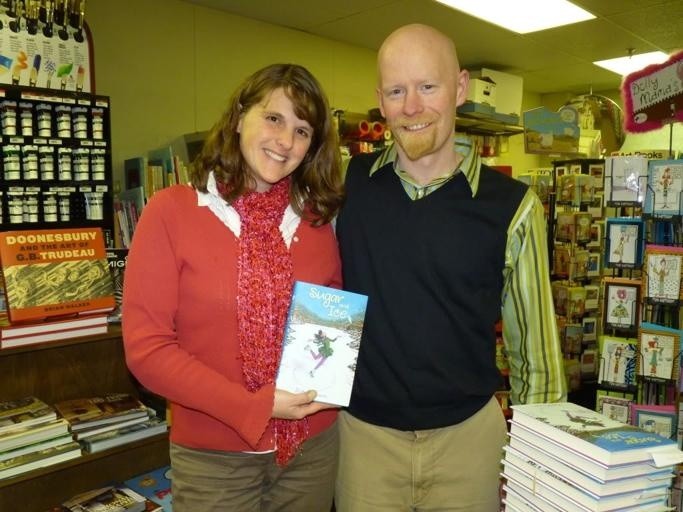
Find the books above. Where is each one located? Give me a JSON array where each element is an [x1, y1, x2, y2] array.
[[0, 396, 167, 479], [0, 227, 116, 349], [500, 402, 683, 512], [518, 155, 682, 441], [456, 68, 523, 127], [273, 279, 371, 409], [114, 145, 191, 248], [49, 465, 172, 512]]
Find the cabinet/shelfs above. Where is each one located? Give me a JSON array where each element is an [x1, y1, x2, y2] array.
[[327, 108, 682, 510], [0, 83, 176, 512]]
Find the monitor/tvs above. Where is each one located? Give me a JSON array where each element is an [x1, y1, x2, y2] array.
[[273, 279, 371, 409]]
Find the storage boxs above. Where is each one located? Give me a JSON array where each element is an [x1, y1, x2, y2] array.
[[456, 68, 523, 127]]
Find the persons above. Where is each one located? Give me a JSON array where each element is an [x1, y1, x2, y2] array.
[[120, 62, 342, 510], [304, 330, 339, 378], [337, 19, 570, 512]]
